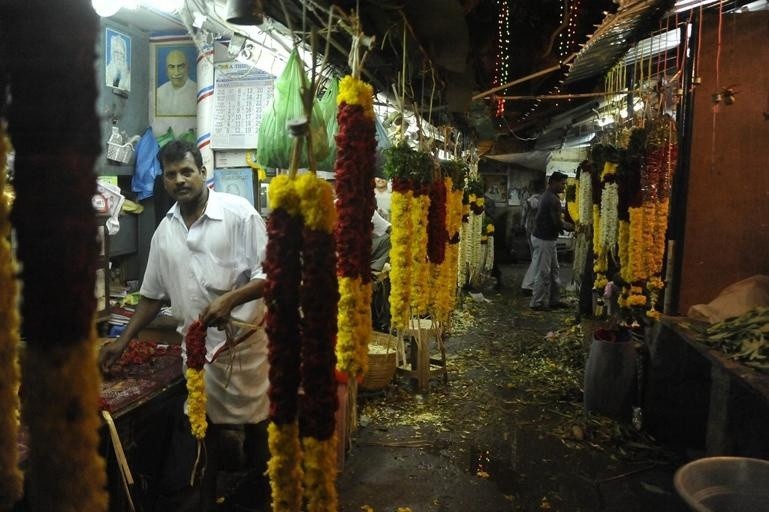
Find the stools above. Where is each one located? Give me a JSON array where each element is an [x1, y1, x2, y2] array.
[[397, 317, 448, 395]]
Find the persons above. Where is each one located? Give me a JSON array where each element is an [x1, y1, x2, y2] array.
[[528, 171, 575, 312], [156, 49, 197, 116], [95, 139, 272, 512], [370, 210, 393, 272], [106, 35, 130, 91], [520, 183, 541, 296]]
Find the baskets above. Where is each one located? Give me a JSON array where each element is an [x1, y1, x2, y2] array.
[[358, 331, 400, 390], [106, 134, 136, 164]]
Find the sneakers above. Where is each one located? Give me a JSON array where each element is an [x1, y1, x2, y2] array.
[[520, 289, 532, 296], [530, 302, 567, 312]]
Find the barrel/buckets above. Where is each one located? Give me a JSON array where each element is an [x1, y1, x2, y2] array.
[[583, 329, 639, 425], [671, 457, 768, 512]]
